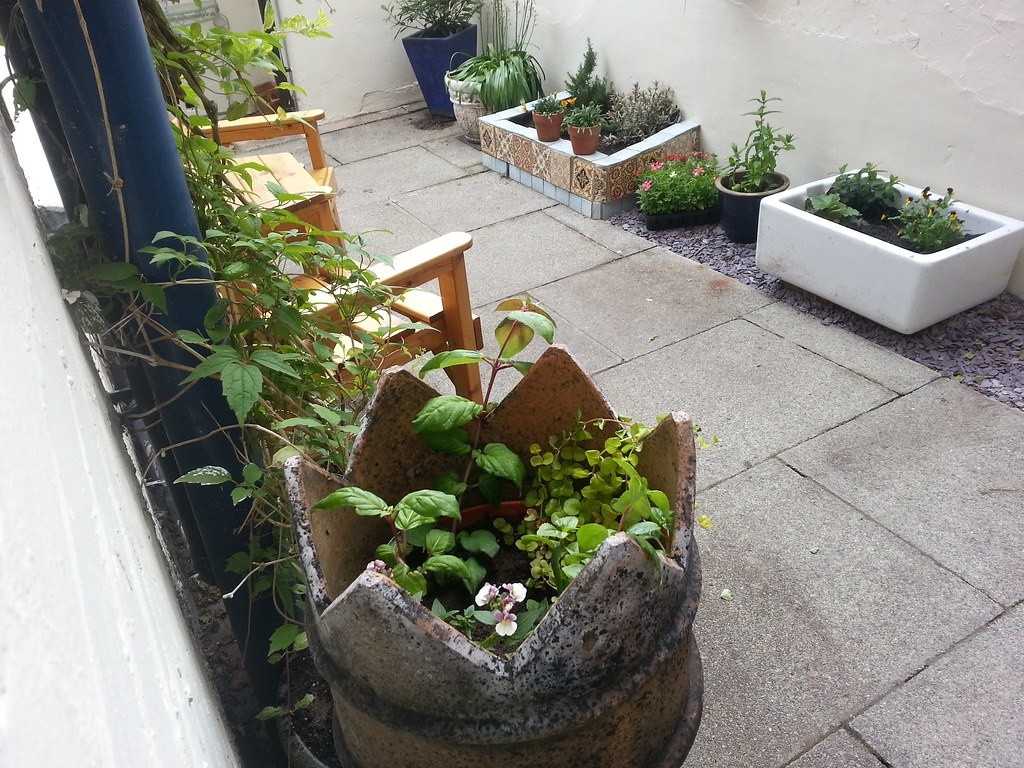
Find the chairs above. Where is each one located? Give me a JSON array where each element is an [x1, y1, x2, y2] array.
[[192, 230, 484, 454], [163, 109, 346, 254]]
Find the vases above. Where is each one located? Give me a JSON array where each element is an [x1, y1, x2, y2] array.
[[755, 161, 1024, 338], [645, 206, 718, 232]]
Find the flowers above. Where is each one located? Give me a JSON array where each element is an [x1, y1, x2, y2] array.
[[799, 159, 973, 251], [631, 151, 721, 214]]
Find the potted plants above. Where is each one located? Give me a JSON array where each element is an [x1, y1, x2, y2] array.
[[562, 99, 603, 156], [476, 37, 702, 222], [530, 91, 568, 142], [279, 288, 707, 768], [379, 0, 483, 122], [443, 0, 547, 146], [715, 90, 800, 246]]
